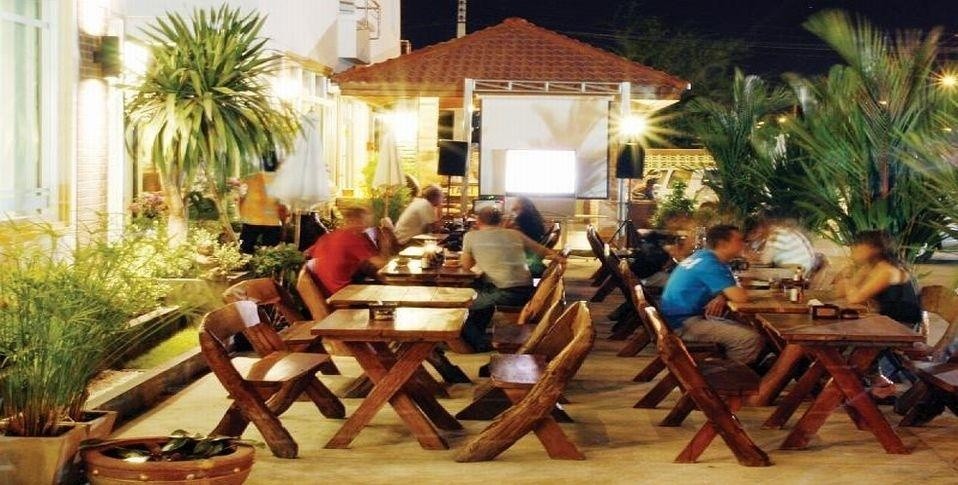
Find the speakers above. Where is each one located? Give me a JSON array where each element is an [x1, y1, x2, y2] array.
[[437, 139, 469, 178], [614, 142, 645, 180]]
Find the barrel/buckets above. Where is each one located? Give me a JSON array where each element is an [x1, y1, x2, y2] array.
[[574, 196, 590, 225], [589, 197, 609, 227]]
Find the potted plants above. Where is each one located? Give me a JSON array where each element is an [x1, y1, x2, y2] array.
[[84, 429, 267, 485], [0, 201, 251, 485]]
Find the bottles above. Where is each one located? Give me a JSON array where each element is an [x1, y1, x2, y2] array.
[[419, 239, 432, 272], [790, 266, 803, 303]]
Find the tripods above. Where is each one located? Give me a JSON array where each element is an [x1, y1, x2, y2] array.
[[594, 180, 642, 260]]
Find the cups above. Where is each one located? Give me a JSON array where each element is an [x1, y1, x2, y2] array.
[[398, 255, 409, 267], [442, 249, 465, 269]]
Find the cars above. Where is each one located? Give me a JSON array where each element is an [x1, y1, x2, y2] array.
[[641, 167, 724, 202], [915, 214, 958, 264]]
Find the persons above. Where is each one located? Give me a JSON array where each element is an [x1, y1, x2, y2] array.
[[303, 205, 397, 311], [462, 206, 567, 352], [227, 148, 291, 253], [394, 184, 442, 247], [632, 232, 673, 288], [742, 217, 821, 279], [658, 225, 766, 364], [833, 230, 928, 414], [511, 197, 546, 257]]
